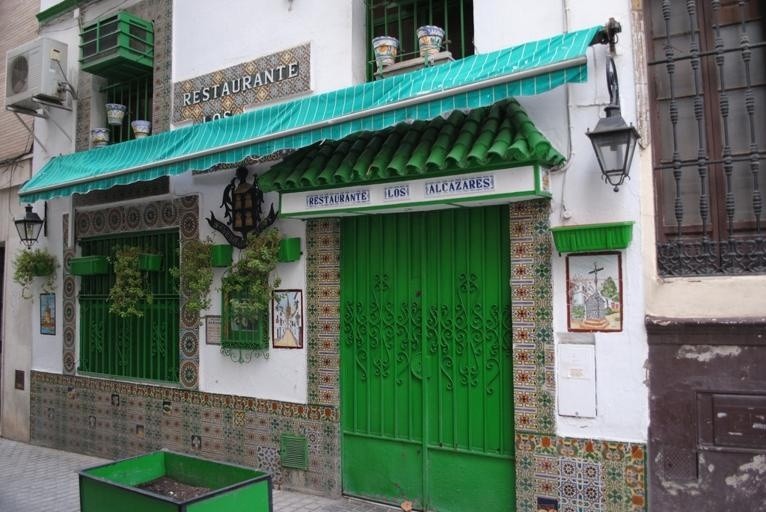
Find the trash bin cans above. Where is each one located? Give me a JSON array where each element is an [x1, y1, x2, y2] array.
[[80, 449, 273, 511]]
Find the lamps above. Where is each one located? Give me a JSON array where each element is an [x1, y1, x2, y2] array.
[[585, 56, 641, 192], [206, 168, 278, 249], [14, 202, 48, 250]]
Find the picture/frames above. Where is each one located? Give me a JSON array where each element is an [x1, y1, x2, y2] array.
[[272, 289, 304, 349], [39, 293, 56, 335], [566, 251, 623, 332]]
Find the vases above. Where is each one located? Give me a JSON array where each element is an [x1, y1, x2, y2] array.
[[416, 26, 445, 57], [372, 35, 400, 67], [69, 256, 108, 276], [91, 103, 152, 148], [549, 221, 633, 257]]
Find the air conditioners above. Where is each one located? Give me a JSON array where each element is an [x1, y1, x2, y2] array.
[[5, 37, 69, 112]]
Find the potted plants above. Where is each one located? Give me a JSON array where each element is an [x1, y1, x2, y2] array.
[[167, 236, 233, 315], [215, 228, 301, 328], [11, 248, 59, 299], [105, 244, 163, 319]]
